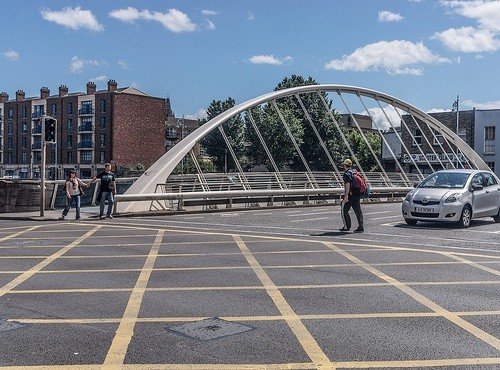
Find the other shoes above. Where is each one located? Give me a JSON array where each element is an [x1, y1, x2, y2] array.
[[62, 211, 64, 219], [107, 213, 113, 219], [99, 217, 105, 220], [77, 218, 83, 220]]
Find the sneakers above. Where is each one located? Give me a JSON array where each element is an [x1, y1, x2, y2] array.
[[339, 227, 350, 231], [354, 226, 364, 232]]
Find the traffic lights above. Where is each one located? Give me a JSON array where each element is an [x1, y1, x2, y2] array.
[[45, 117, 56, 144]]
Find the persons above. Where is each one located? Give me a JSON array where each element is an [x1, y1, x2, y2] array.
[[339, 159, 368, 233], [58, 170, 90, 220], [90, 163, 117, 220]]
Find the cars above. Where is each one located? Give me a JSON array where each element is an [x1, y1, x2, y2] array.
[[402, 169, 500, 228]]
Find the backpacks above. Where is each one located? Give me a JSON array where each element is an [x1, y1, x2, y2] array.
[[346, 169, 366, 195]]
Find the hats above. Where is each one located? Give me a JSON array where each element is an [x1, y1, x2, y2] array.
[[342, 159, 352, 166]]
